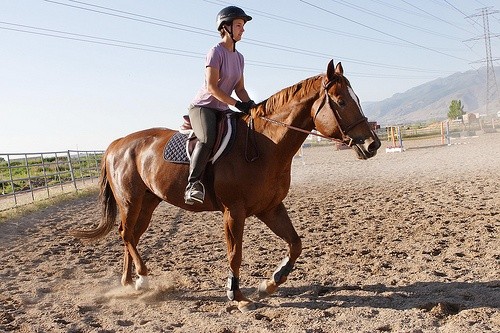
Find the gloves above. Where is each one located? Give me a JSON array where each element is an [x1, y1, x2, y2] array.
[[235, 100, 255, 115]]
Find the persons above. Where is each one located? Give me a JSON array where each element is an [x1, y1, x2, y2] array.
[[184, 5, 257, 205]]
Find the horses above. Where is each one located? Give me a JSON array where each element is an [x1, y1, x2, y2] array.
[[71, 58, 382, 315]]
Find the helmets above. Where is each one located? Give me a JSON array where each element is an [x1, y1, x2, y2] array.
[[217, 6, 253, 32]]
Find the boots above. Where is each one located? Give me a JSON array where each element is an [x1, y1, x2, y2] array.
[[184, 140, 212, 204]]
